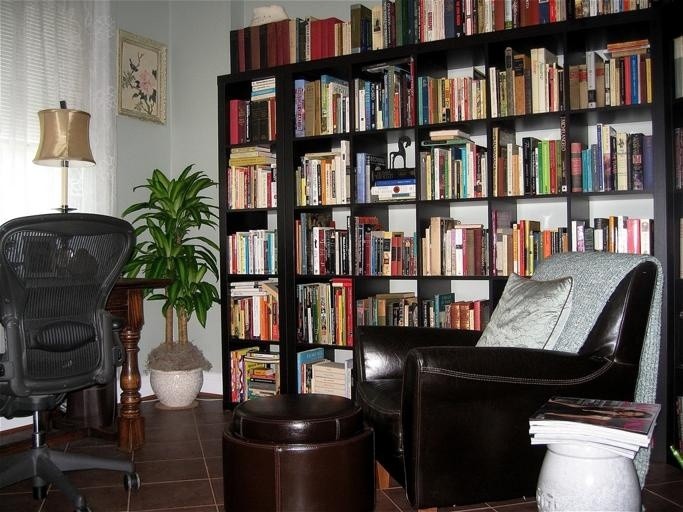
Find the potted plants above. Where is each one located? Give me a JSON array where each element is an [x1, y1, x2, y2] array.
[[120, 163, 219, 412]]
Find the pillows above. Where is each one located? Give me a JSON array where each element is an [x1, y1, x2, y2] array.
[[474, 273, 573, 351]]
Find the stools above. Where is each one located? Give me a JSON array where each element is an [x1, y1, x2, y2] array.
[[221, 392, 374, 510]]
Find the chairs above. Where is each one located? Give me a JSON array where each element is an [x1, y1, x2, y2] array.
[[0, 213, 142, 511], [355, 251, 663, 512]]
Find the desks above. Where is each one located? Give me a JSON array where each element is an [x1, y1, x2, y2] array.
[[535, 443, 643, 510], [22, 277, 175, 454]]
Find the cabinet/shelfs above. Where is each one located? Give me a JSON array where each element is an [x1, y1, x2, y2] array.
[[217, 2, 676, 462]]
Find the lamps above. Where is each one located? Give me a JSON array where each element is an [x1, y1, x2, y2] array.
[[33, 100, 97, 214]]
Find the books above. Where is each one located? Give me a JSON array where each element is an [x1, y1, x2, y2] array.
[[227, 142, 416, 277], [229, 275, 494, 403], [417, 116, 651, 278], [418, 2, 653, 121], [230, 2, 417, 145], [527, 396, 663, 460]]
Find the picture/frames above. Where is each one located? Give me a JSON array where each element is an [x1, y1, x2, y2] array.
[[21, 235, 55, 279], [116, 29, 168, 126]]
